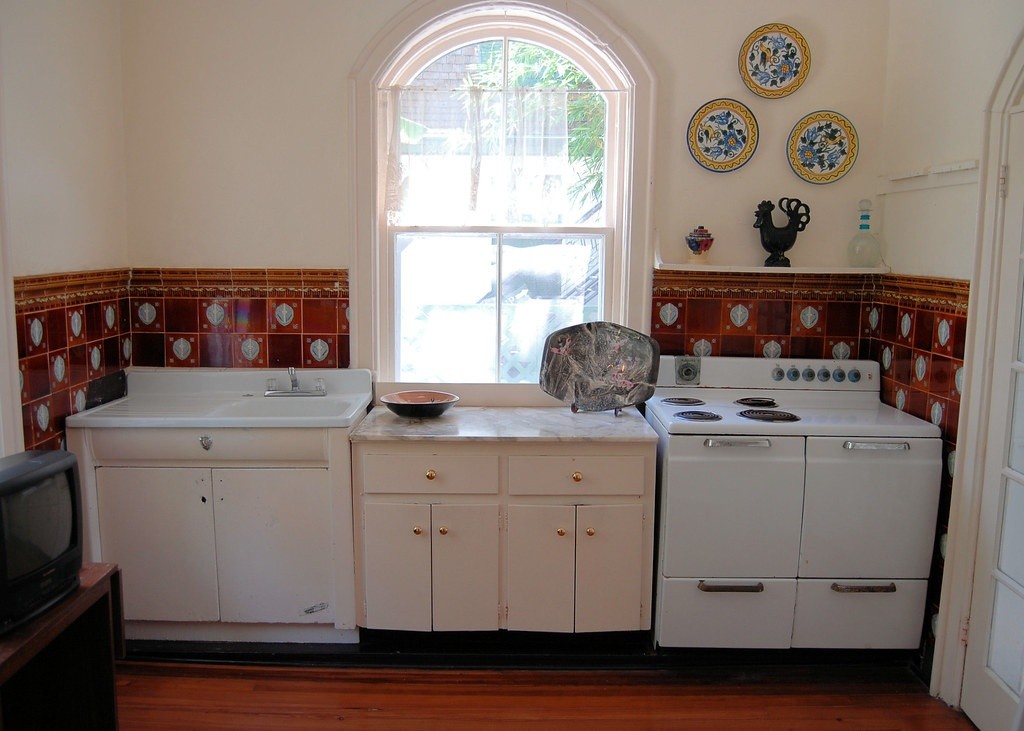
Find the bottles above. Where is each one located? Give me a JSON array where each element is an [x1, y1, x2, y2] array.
[[847, 199, 880, 267]]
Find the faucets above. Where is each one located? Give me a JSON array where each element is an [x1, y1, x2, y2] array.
[[287, 367, 300, 392]]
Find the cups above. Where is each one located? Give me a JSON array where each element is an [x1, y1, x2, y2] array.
[[685, 225, 715, 264]]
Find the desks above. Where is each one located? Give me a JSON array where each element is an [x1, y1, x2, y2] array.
[[0, 561, 124, 731]]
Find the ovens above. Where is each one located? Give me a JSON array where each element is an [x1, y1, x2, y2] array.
[[643, 407, 944, 654]]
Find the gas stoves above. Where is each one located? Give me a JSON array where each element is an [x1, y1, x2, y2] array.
[[646, 354, 944, 431]]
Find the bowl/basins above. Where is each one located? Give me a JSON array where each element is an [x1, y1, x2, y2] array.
[[380, 391, 460, 419]]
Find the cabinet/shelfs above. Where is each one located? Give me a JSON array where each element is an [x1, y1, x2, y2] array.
[[65, 426, 363, 645], [350, 441, 660, 636]]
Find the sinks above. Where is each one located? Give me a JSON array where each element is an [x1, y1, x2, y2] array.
[[207, 395, 353, 418]]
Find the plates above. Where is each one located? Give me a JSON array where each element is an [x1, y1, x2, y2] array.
[[687, 98, 759, 173], [738, 23, 811, 99], [786, 110, 860, 185]]
